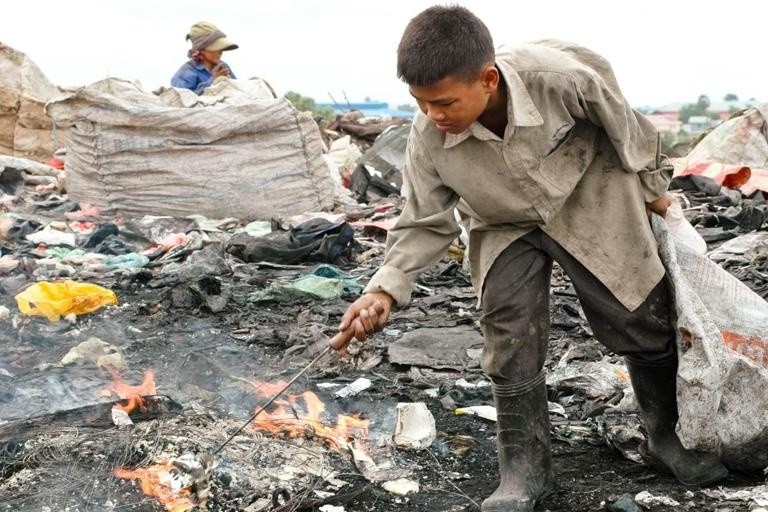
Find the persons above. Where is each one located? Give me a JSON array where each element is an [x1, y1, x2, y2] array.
[[170, 20, 239, 96], [334, 5, 728, 512]]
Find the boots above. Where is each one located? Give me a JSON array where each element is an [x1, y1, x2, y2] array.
[[624, 354, 729, 487], [481, 370, 556, 512]]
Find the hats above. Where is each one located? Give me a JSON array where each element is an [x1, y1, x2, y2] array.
[[187, 22, 239, 57]]
